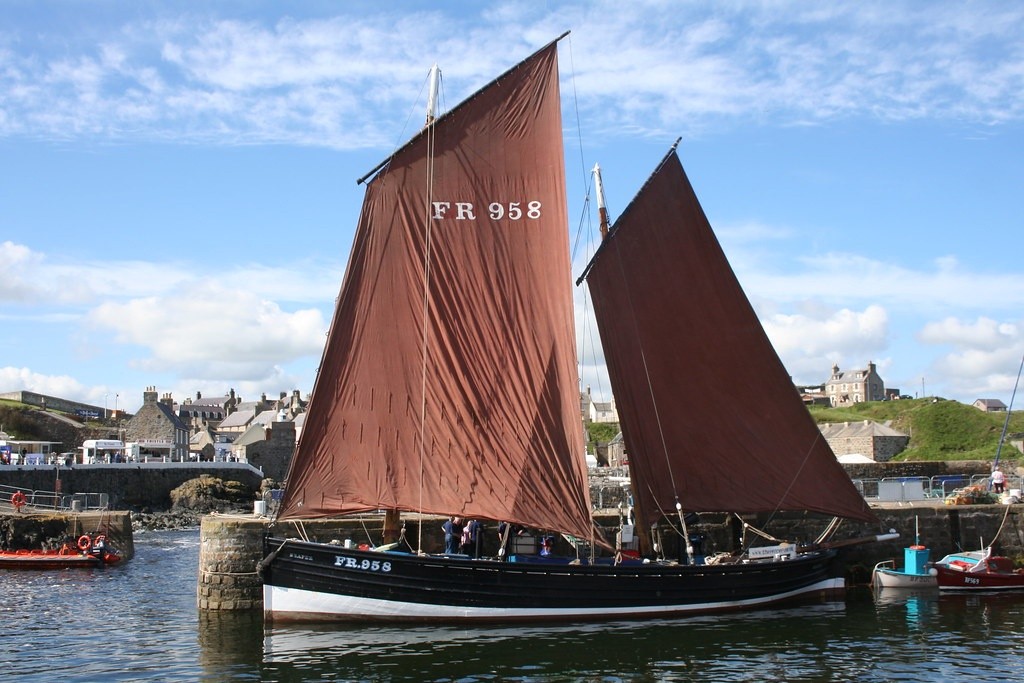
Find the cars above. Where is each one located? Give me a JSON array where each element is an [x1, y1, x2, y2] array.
[[900, 394, 913, 400]]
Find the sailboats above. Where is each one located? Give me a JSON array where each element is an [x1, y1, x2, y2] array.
[[257, 22, 902, 627]]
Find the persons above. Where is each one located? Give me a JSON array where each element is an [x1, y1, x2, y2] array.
[[499, 521, 511, 557], [453, 517, 463, 552], [61, 531, 70, 542], [470, 519, 483, 556], [542, 535, 549, 554], [115, 452, 121, 463], [22, 447, 27, 457], [462, 519, 472, 554], [992, 467, 1004, 492], [442, 516, 460, 555]]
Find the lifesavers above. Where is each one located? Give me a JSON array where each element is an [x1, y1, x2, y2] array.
[[78, 535, 91, 550], [11, 493, 25, 507], [95, 535, 107, 547]]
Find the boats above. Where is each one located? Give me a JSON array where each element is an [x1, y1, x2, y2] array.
[[929, 536, 1024, 592], [0, 512, 121, 568], [874, 514, 939, 588]]
